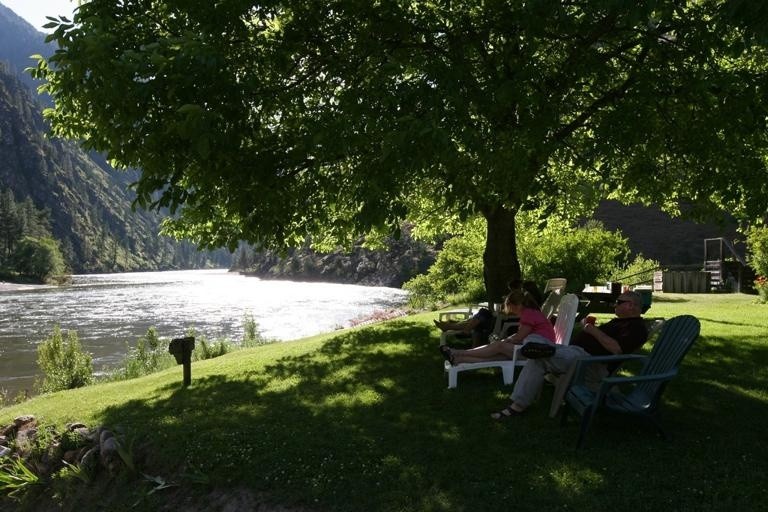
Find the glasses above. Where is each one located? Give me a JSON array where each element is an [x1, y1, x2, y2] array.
[[616, 299, 631, 305]]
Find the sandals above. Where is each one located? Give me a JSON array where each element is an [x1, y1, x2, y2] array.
[[520, 342, 556, 359], [490, 406, 526, 420]]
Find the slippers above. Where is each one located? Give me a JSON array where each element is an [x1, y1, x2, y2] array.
[[440, 345, 458, 366]]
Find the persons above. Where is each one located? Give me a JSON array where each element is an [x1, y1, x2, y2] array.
[[489, 290, 646, 419], [433, 279, 556, 367]]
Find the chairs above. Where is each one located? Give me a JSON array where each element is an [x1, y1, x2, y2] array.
[[439, 305, 488, 321], [443, 294, 579, 389], [488, 291, 562, 345], [536, 318, 665, 418], [561, 315, 700, 451], [544, 278, 568, 293]]
